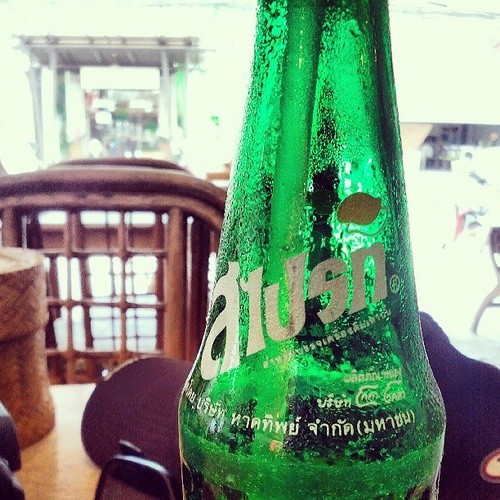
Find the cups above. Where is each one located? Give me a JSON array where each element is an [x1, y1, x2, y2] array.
[[0, 245, 53, 448]]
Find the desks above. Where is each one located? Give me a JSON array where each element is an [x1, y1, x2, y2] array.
[[12, 383, 135, 500]]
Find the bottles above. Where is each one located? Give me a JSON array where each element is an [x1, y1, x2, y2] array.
[[178, 0, 447, 500]]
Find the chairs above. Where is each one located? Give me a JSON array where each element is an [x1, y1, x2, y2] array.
[[0, 157, 227, 385], [469, 226, 500, 334]]
